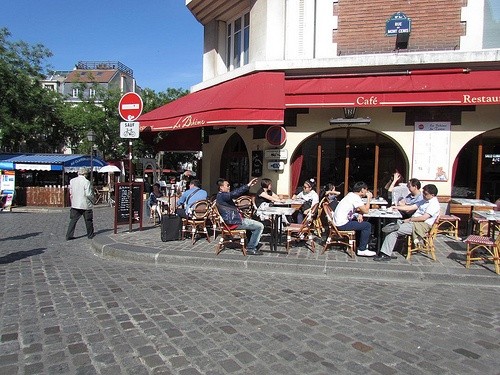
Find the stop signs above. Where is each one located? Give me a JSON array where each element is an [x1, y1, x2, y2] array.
[[119, 92, 143, 122]]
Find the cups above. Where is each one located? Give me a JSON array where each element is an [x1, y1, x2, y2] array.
[[265, 203, 269, 207], [366, 193, 372, 196], [379, 197, 383, 202], [381, 206, 386, 210], [387, 208, 392, 212]]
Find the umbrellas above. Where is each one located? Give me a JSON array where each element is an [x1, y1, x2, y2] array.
[[98, 165, 121, 173]]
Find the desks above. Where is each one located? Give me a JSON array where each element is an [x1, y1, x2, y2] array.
[[362, 198, 388, 209], [264, 205, 296, 251], [98, 191, 109, 203], [449, 198, 498, 236], [156, 197, 179, 214], [471, 211, 500, 245], [273, 199, 304, 220], [362, 208, 402, 260]]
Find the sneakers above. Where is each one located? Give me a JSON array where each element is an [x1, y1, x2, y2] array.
[[357, 249, 376, 256]]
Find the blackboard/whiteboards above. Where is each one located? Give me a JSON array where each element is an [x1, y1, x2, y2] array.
[[115, 182, 143, 225]]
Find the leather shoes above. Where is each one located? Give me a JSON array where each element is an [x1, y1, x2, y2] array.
[[381, 224, 399, 233], [245, 250, 263, 255], [374, 252, 391, 261]]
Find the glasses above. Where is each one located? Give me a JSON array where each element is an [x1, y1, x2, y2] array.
[[302, 186, 311, 189]]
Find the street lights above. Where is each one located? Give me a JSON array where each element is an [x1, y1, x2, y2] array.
[[87, 129, 97, 195]]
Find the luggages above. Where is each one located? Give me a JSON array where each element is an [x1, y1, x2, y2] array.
[[161, 189, 183, 242]]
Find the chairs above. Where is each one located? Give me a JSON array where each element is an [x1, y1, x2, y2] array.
[[286, 204, 316, 253], [323, 204, 357, 258], [437, 211, 460, 242], [147, 199, 160, 226], [463, 227, 500, 275], [309, 196, 326, 237], [182, 197, 256, 256], [400, 213, 440, 262]]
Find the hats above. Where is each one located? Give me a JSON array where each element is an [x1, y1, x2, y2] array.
[[78, 168, 88, 174]]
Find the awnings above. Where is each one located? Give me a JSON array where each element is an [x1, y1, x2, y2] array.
[[133, 71, 286, 155]]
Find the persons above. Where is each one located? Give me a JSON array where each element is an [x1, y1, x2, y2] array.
[[332, 181, 377, 256], [292, 178, 319, 239], [374, 184, 440, 262], [384, 172, 411, 205], [149, 183, 162, 223], [216, 178, 264, 255], [256, 177, 290, 231], [176, 180, 208, 221], [390, 178, 427, 220], [321, 183, 341, 211], [66, 168, 97, 240]]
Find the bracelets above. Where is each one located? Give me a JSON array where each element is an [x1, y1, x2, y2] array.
[[409, 218, 411, 221]]
